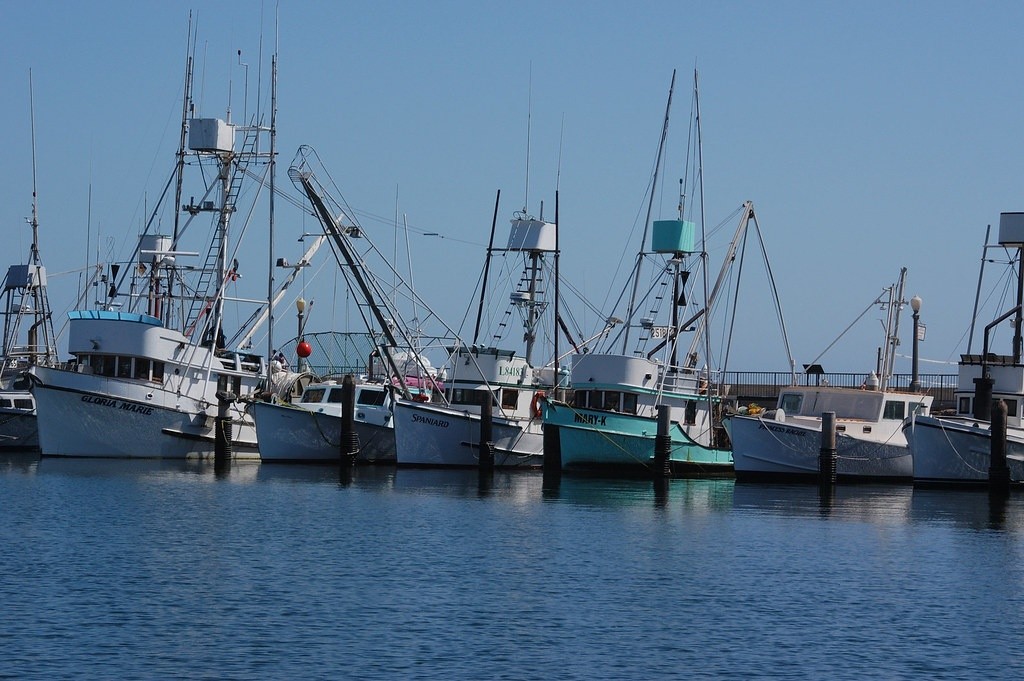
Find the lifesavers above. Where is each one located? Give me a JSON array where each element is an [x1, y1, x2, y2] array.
[[532, 391, 545, 417]]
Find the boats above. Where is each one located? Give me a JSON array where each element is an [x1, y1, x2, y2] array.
[[0, 0, 559, 477], [534, 61, 794, 483], [726, 265, 959, 486], [902, 210, 1024, 491]]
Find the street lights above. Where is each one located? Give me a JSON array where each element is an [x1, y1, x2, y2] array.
[[296, 296, 307, 373], [909, 294, 923, 395]]
